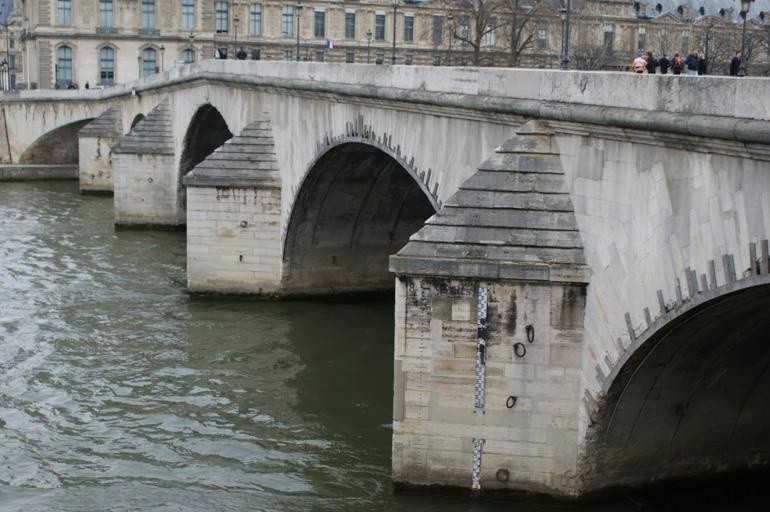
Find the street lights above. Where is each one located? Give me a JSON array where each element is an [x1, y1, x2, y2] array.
[[160, 44, 166, 72], [295, 0, 303, 62], [736, 0, 755, 77], [188, 31, 194, 61], [446, 9, 455, 66], [559, 6, 568, 68], [0, 15, 17, 92], [233, 14, 240, 56], [365, 29, 372, 63]]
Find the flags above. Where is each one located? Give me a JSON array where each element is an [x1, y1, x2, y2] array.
[[326, 38, 336, 49]]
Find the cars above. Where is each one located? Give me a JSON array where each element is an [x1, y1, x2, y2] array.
[[55, 78, 78, 89], [92, 79, 115, 90]]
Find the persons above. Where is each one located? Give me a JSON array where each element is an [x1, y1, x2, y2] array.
[[85, 80, 90, 89], [236, 47, 247, 60], [729, 51, 743, 76], [632, 48, 708, 75], [217, 48, 224, 58]]
